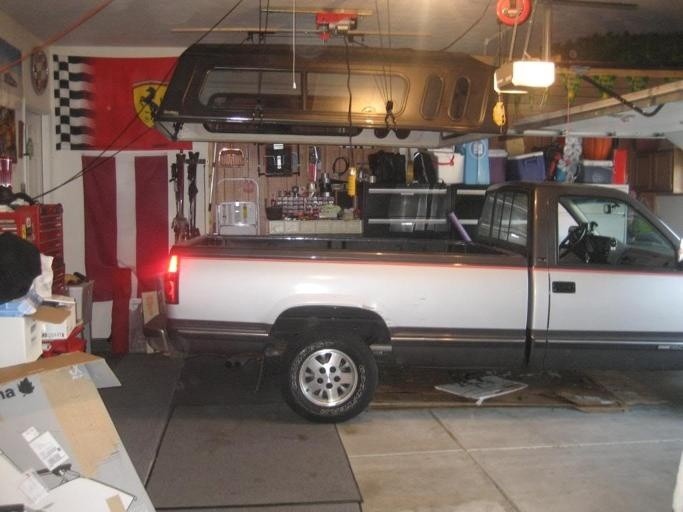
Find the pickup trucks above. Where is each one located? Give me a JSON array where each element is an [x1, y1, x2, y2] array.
[[141, 182, 683, 423]]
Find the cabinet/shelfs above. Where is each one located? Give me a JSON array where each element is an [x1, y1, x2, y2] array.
[[627, 151, 681, 198], [366, 186, 481, 243]]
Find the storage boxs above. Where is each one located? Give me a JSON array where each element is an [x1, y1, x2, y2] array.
[[43, 301, 77, 341], [507, 150, 548, 189], [467, 133, 489, 186], [0, 307, 65, 365], [581, 161, 620, 192], [3, 349, 157, 512], [487, 146, 502, 185], [501, 137, 520, 152], [435, 152, 465, 190]]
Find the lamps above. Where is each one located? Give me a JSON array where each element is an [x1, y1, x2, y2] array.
[[491, 57, 556, 95]]
